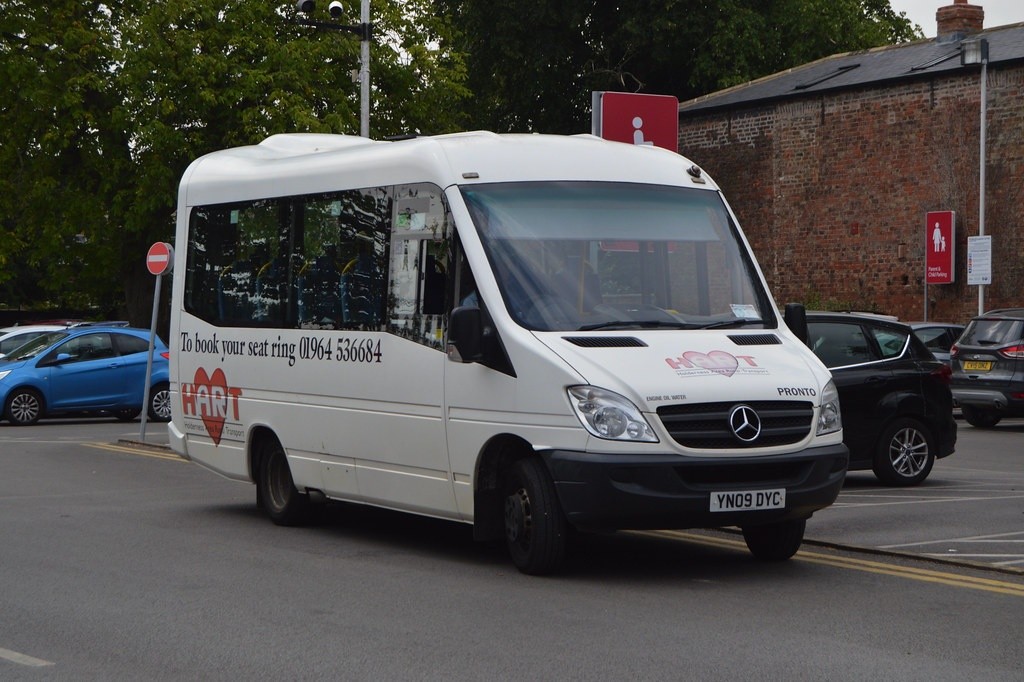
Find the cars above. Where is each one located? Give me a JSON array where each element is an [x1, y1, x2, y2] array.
[[0, 318, 133, 356], [853, 321, 969, 366], [0, 324, 169, 428]]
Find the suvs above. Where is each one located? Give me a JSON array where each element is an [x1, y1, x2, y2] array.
[[774, 309, 959, 487], [949, 306, 1024, 427]]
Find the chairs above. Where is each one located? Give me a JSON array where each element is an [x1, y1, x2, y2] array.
[[217, 261, 251, 321], [297, 259, 340, 322], [257, 259, 291, 320], [342, 257, 387, 325], [571, 257, 602, 313]]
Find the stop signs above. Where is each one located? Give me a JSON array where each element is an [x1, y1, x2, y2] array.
[[147, 242, 171, 274]]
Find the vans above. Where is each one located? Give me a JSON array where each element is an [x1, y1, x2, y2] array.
[[167, 126, 850, 575]]
